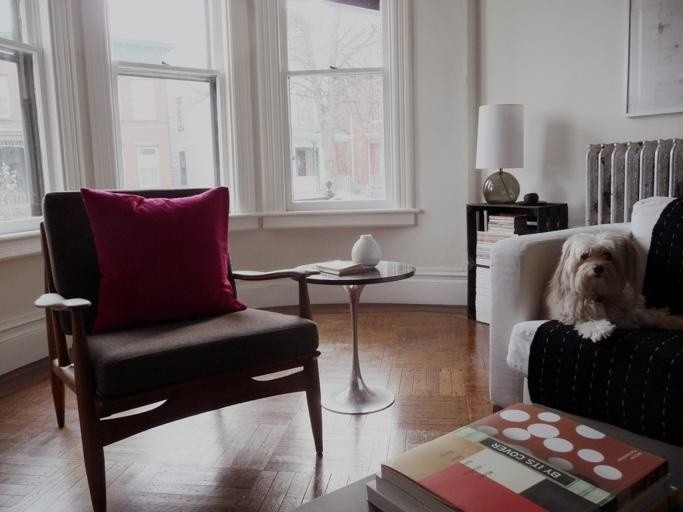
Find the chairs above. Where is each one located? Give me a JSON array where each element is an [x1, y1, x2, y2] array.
[[33, 187, 322, 511]]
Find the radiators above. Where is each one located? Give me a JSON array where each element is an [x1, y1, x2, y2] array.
[[585, 139, 683, 226]]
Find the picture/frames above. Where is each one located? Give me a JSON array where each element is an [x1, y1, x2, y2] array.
[[623, 0, 682, 119]]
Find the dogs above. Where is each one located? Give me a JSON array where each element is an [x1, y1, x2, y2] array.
[[539, 226, 683, 343]]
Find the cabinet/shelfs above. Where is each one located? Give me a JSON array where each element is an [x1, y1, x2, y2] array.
[[467, 203, 568, 325]]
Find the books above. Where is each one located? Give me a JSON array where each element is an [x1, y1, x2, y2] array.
[[315, 260, 363, 276], [474, 214, 527, 325], [375, 472, 431, 512], [381, 402, 669, 512], [366, 480, 407, 511]]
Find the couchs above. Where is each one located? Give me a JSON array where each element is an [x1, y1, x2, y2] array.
[[488, 196, 682, 446]]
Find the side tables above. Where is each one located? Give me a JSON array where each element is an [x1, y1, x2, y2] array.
[[291, 261, 414, 414]]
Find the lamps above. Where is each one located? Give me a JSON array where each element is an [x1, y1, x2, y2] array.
[[476, 104, 524, 202]]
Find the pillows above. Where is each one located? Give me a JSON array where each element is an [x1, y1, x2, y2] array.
[[80, 188, 247, 336]]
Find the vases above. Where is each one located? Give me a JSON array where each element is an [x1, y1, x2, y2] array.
[[350, 233, 383, 269]]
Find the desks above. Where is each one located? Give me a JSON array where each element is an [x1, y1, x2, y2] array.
[[287, 404, 681, 511]]
